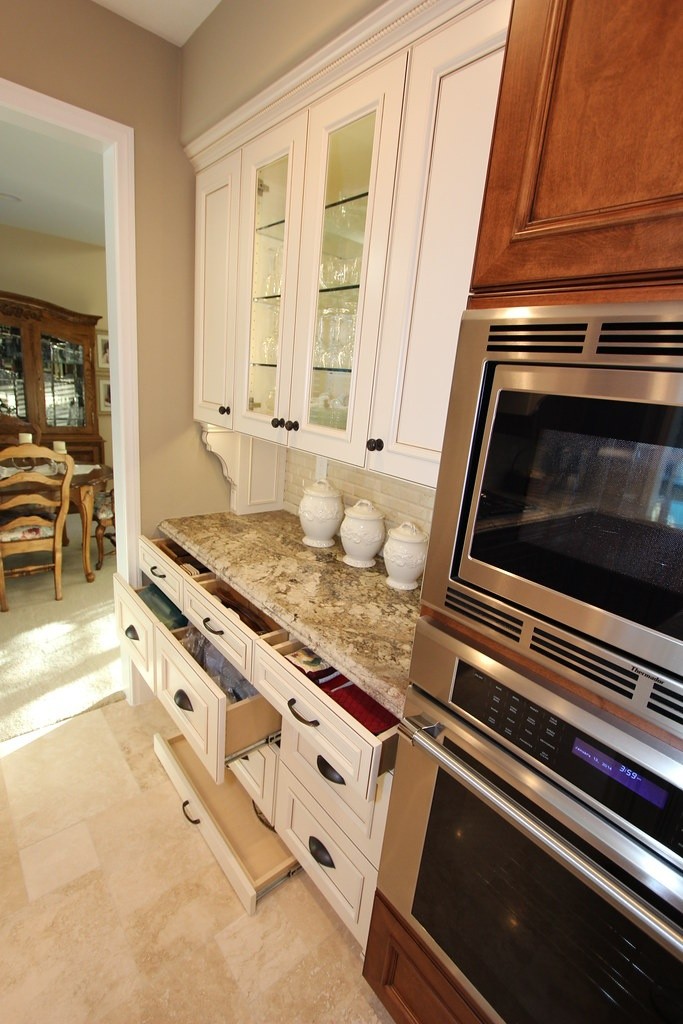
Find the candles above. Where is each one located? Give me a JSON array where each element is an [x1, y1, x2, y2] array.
[[18, 432, 32, 444], [53, 441, 65, 453]]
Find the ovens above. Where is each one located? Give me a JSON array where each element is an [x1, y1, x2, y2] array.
[[361, 301, 683, 1024]]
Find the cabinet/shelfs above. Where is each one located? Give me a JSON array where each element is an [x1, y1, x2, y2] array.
[[0, 435, 106, 516], [232, 51, 409, 469], [113, 532, 399, 955], [363, 0, 513, 489], [194, 147, 244, 431], [468, 0, 683, 312], [0, 289, 107, 515]]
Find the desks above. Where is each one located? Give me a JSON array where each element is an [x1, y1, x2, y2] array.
[[0, 460, 114, 583]]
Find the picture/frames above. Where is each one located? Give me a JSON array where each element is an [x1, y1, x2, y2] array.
[[94, 329, 112, 416]]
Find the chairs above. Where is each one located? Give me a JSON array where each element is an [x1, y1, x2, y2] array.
[[0, 444, 76, 613], [92, 488, 115, 569]]
